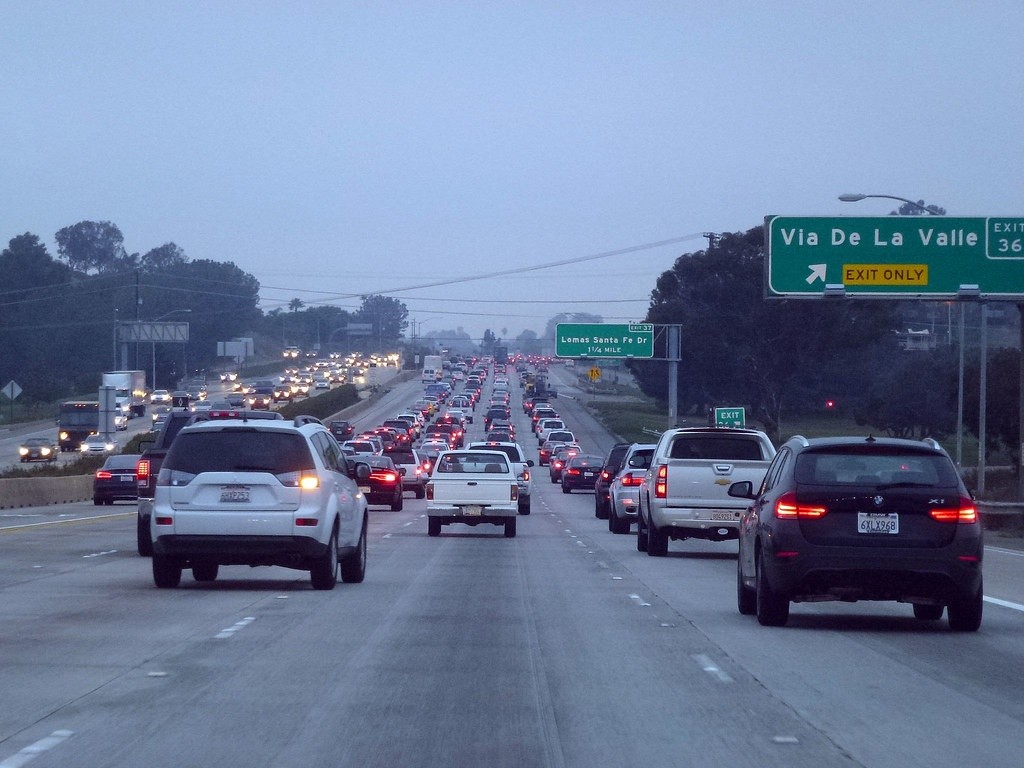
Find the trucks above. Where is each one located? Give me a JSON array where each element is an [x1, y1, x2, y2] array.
[[100, 372, 150, 419], [60, 402, 100, 450]]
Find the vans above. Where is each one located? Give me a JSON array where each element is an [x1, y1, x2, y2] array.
[[424, 355, 444, 369]]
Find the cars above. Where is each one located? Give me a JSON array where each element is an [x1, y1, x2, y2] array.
[[79, 435, 119, 456], [114, 416, 129, 430], [150, 389, 214, 423], [329, 352, 606, 538], [18, 437, 59, 462], [217, 346, 402, 411]]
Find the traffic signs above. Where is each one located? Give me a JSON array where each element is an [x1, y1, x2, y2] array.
[[763, 213, 1024, 296], [554, 320, 657, 360], [554, 323, 654, 359]]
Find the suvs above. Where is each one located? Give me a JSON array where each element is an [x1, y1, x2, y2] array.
[[727, 434, 987, 633], [93, 410, 372, 589], [596, 426, 778, 557]]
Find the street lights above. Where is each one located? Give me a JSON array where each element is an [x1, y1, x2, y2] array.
[[837, 194, 986, 493], [151, 308, 193, 391]]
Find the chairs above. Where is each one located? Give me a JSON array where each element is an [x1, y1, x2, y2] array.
[[452, 464, 464, 471], [817, 472, 837, 482], [856, 475, 880, 483], [893, 471, 926, 483], [485, 464, 502, 472]]
